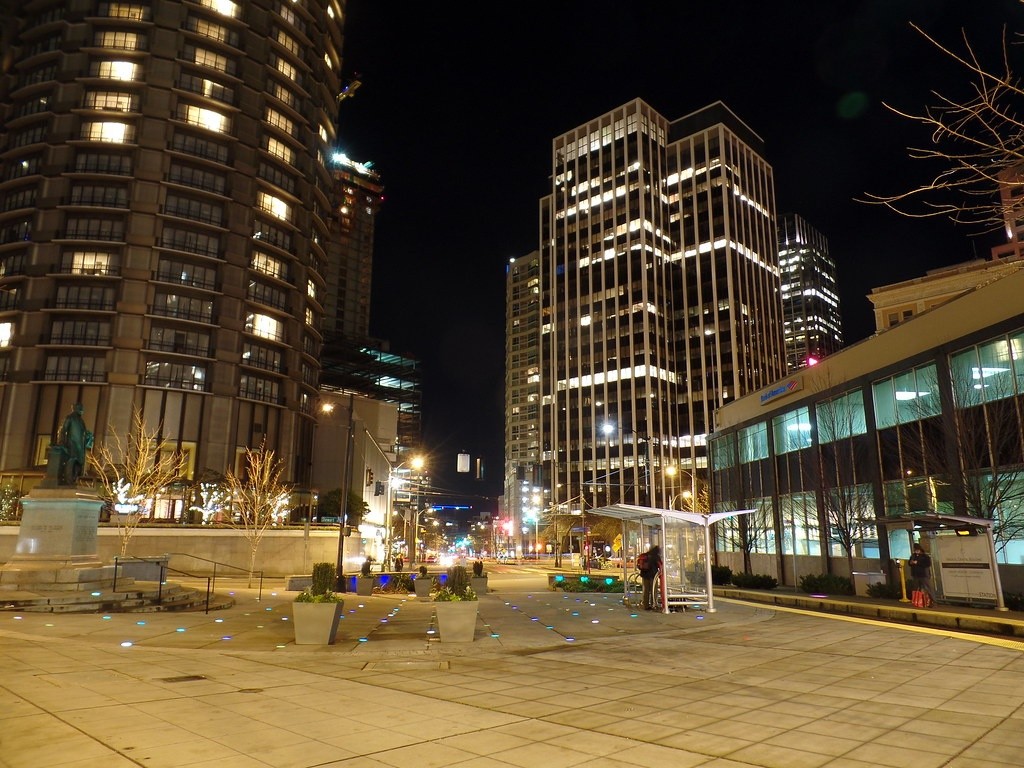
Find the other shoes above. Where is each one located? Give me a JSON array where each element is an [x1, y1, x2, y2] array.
[[652, 606, 661, 611]]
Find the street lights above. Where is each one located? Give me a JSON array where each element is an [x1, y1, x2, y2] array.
[[502, 520, 513, 559], [603, 425, 656, 510], [556, 483, 585, 568], [383, 457, 438, 571], [320, 394, 355, 594]]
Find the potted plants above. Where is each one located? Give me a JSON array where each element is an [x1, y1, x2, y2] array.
[[431, 566, 479, 643], [412, 565, 431, 597], [355, 558, 376, 596], [470, 561, 488, 597], [292, 562, 344, 645]]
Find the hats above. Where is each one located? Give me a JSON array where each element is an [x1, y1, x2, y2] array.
[[914, 543, 920, 550]]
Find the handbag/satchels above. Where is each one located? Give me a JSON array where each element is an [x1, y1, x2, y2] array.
[[912, 588, 932, 607]]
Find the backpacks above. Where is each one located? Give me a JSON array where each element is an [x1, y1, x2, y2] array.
[[637, 553, 653, 571]]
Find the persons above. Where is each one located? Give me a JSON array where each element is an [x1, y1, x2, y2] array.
[[640, 546, 665, 611], [58, 401, 94, 486], [908, 543, 938, 608], [395, 553, 404, 572]]
